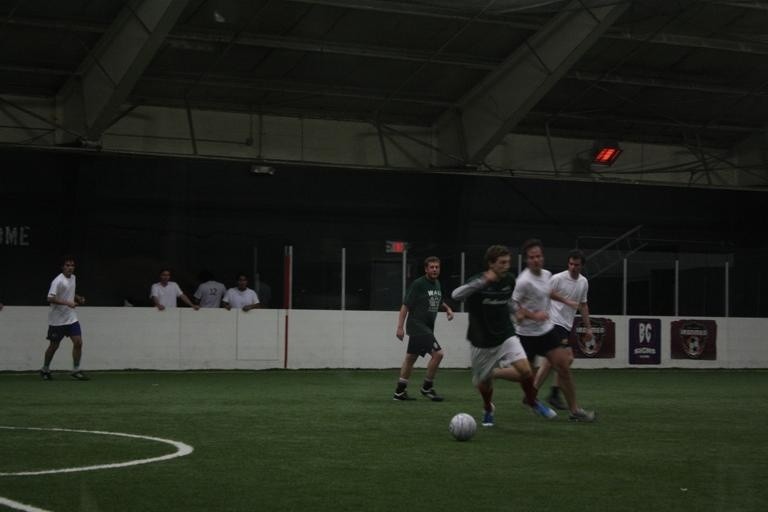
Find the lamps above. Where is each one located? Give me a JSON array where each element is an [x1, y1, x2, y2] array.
[[589, 138, 623, 167]]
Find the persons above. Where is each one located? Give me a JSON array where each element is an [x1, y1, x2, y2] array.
[[522, 249, 593, 409], [393, 256, 453, 401], [193, 272, 228, 308], [221, 274, 263, 311], [41, 255, 90, 381], [451, 244, 558, 427], [149, 269, 199, 310], [0, 302, 4, 312], [509, 238, 597, 422]]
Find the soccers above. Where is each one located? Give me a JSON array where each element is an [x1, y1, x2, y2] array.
[[449, 413, 477, 441]]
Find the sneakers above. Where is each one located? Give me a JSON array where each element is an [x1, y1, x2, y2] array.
[[522, 398, 529, 406], [481, 404, 496, 428], [71, 371, 89, 380], [544, 388, 569, 410], [392, 391, 416, 401], [41, 367, 50, 380], [419, 385, 444, 402], [569, 408, 597, 422], [533, 402, 557, 421]]
